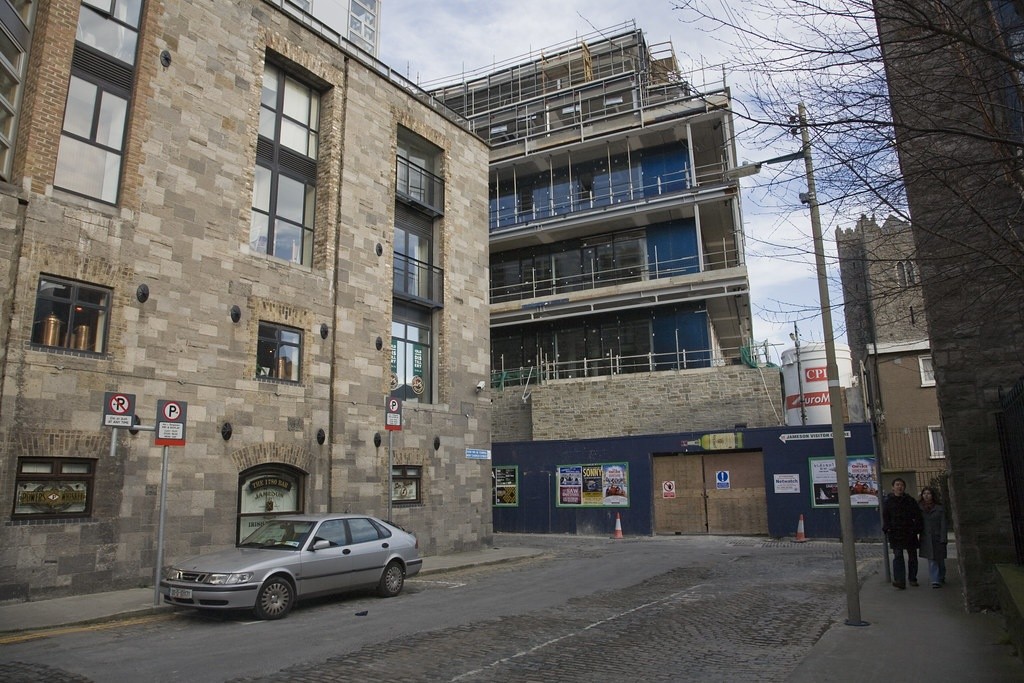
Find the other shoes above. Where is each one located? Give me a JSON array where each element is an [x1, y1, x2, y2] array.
[[932, 583, 940, 588], [893, 580, 906, 590], [938, 579, 945, 584], [909, 581, 919, 586]]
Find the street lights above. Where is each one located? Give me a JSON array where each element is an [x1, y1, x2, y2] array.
[[722, 101, 863, 628]]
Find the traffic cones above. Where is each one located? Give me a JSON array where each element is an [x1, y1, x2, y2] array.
[[791, 514, 810, 543], [610, 512, 626, 539]]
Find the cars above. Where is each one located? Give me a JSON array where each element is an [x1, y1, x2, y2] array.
[[158, 512, 422, 620]]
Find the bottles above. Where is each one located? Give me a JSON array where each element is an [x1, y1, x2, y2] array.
[[681, 432, 743, 451]]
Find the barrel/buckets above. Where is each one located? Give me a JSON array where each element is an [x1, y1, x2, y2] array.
[[42, 311, 60, 346], [278, 356, 286, 379], [76, 322, 90, 350]]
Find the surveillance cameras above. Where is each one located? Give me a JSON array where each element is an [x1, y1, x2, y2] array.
[[477, 381, 485, 390]]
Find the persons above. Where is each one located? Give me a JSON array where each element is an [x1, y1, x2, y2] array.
[[882, 478, 924, 590], [918, 487, 948, 589]]
